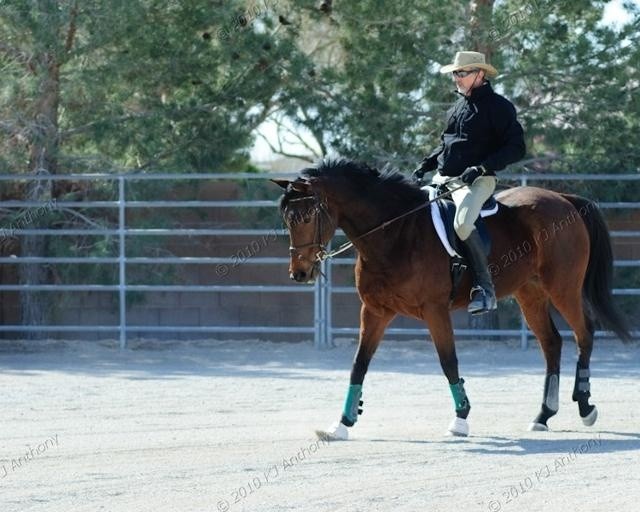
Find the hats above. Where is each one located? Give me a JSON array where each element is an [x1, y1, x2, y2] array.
[[439, 51, 498, 78]]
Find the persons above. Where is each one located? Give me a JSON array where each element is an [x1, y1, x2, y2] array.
[[411, 52, 526, 314]]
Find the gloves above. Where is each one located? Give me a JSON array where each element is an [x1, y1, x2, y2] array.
[[411, 161, 432, 185], [457, 166, 483, 186]]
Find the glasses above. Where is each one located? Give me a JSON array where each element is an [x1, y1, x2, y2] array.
[[452, 70, 479, 77]]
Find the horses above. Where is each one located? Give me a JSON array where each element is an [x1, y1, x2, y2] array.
[[269, 156, 635, 442]]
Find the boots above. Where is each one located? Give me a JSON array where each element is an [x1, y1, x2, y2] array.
[[462, 228, 498, 314]]
